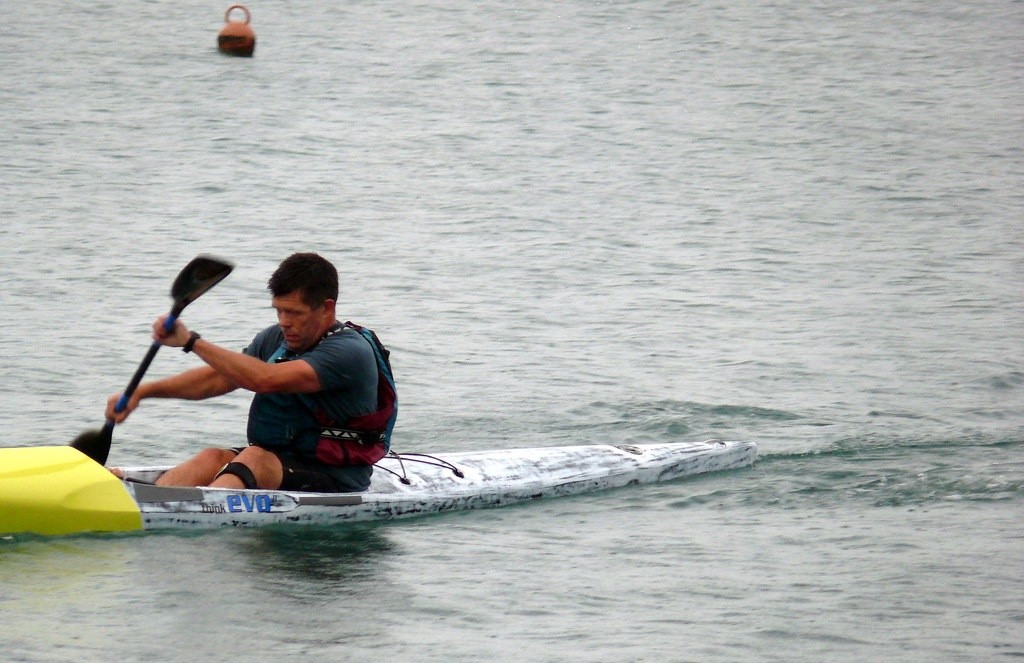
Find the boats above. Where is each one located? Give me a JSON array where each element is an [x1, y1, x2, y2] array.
[[0, 439, 759, 539]]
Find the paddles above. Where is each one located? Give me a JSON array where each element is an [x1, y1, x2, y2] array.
[[68, 252, 236, 467]]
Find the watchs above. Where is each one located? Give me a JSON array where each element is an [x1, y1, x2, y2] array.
[[181, 330, 201, 353]]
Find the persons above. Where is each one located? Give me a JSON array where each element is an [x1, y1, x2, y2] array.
[[104, 252, 378, 494]]
